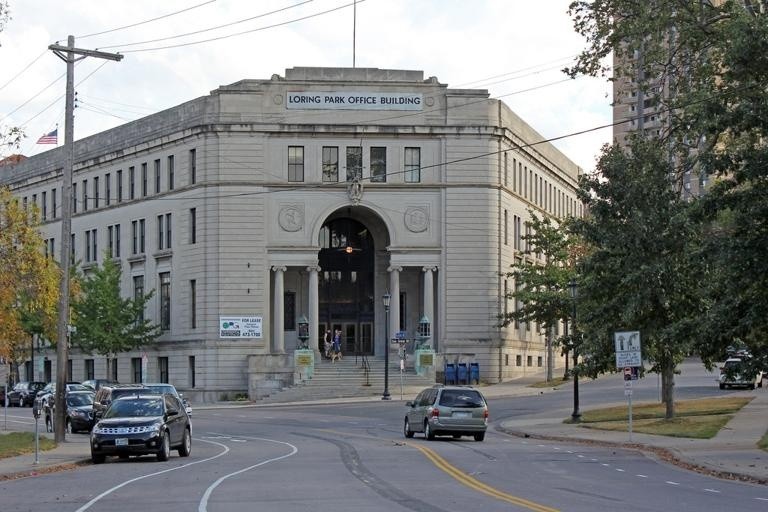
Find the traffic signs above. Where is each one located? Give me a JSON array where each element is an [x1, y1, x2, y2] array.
[[391, 332, 417, 345]]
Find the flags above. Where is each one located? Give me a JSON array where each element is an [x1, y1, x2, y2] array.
[[36, 129, 57, 144]]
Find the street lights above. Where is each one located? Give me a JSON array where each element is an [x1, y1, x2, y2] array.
[[382, 289, 391, 401], [568, 280, 582, 420]]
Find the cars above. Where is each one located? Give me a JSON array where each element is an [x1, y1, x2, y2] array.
[[718, 346, 768, 390], [404, 383, 488, 441], [0, 379, 192, 464]]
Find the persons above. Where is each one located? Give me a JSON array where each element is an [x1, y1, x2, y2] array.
[[324, 329, 342, 361]]
